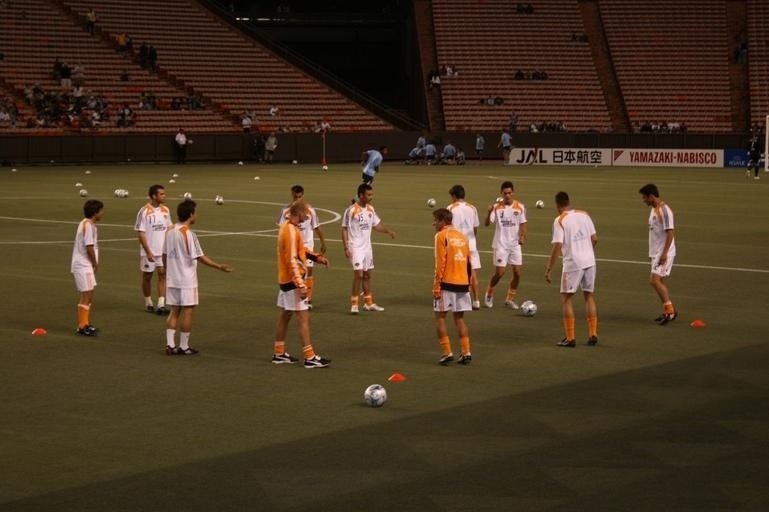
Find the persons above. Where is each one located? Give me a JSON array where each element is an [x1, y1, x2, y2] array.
[[138, 89, 207, 111], [111, 33, 158, 69], [516, 4, 535, 14], [428, 64, 461, 87], [498, 128, 512, 160], [135, 185, 173, 316], [351, 145, 388, 203], [636, 119, 688, 133], [447, 184, 482, 310], [341, 184, 397, 314], [176, 128, 189, 165], [271, 103, 281, 117], [571, 33, 589, 42], [163, 199, 235, 355], [510, 111, 569, 134], [277, 185, 326, 311], [431, 208, 472, 364], [474, 133, 485, 161], [264, 132, 278, 164], [513, 69, 548, 82], [272, 202, 334, 368], [87, 8, 98, 36], [310, 120, 330, 134], [243, 115, 252, 132], [54, 59, 110, 130], [488, 96, 494, 104], [545, 192, 599, 348], [70, 200, 103, 338], [115, 102, 137, 128], [406, 134, 467, 166], [120, 69, 130, 81], [638, 184, 678, 326], [745, 132, 762, 180], [484, 181, 528, 309], [1, 82, 67, 130]]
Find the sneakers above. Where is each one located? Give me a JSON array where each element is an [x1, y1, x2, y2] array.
[[746, 170, 750, 176], [363, 304, 384, 312], [659, 313, 676, 324], [351, 304, 358, 313], [505, 300, 519, 310], [156, 307, 170, 315], [587, 336, 597, 344], [485, 292, 493, 308], [145, 304, 153, 312], [656, 313, 666, 320], [167, 346, 177, 355], [557, 337, 575, 347], [304, 357, 332, 368], [439, 353, 453, 364], [76, 327, 94, 337], [754, 176, 760, 180], [272, 354, 299, 364], [178, 347, 197, 354], [459, 353, 471, 364], [86, 325, 97, 331], [473, 300, 480, 309]]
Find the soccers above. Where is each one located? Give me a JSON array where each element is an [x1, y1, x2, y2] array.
[[536, 200, 544, 208], [427, 198, 437, 208], [184, 192, 192, 200], [292, 160, 296, 165], [119, 190, 127, 198], [237, 161, 242, 166], [79, 190, 87, 197], [114, 189, 121, 196], [364, 384, 387, 407], [322, 165, 328, 172], [496, 197, 504, 202], [521, 301, 537, 317], [84, 171, 90, 174], [215, 196, 223, 204]]
